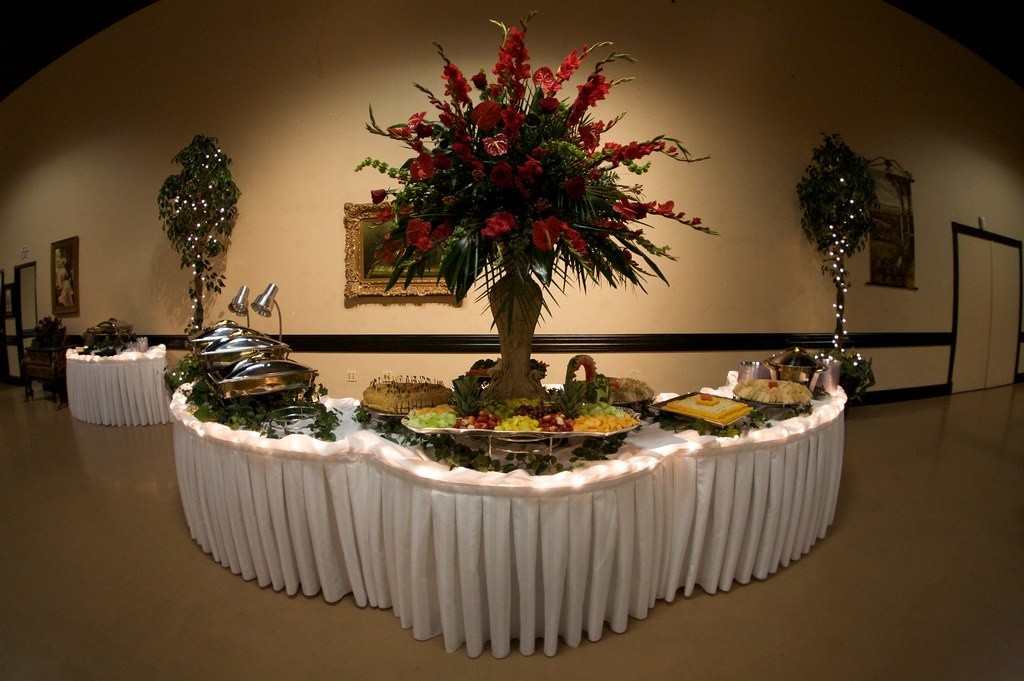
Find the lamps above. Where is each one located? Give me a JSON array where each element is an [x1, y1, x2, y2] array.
[[227, 286, 249, 328], [251, 284, 281, 342]]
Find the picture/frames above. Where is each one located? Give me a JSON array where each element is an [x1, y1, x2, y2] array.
[[50, 236, 80, 316], [342, 199, 468, 300]]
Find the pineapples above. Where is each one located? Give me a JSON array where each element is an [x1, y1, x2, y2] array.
[[573, 416, 638, 432], [494, 415, 542, 431], [416, 404, 454, 415]]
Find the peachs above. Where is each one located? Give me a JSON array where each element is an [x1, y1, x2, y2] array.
[[537, 414, 574, 432], [452, 411, 502, 430]]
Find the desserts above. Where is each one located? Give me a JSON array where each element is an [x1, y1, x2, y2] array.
[[734, 379, 813, 404]]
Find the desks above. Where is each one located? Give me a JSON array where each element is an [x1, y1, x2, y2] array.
[[170, 365, 845, 654], [21, 346, 77, 409], [64, 345, 173, 426]]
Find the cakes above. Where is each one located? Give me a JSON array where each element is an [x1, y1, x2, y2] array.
[[363, 382, 453, 414], [661, 394, 753, 423]]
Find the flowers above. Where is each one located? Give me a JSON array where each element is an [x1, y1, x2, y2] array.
[[33, 316, 69, 341], [368, 9, 716, 300]]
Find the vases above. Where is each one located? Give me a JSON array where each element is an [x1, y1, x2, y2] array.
[[484, 237, 547, 402], [37, 331, 62, 347]]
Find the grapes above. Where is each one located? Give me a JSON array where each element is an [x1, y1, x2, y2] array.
[[487, 404, 555, 419]]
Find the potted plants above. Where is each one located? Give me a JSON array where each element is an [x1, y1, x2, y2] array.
[[156, 133, 242, 398], [798, 131, 873, 399]]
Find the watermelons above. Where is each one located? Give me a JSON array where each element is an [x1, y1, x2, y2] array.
[[565, 355, 598, 384]]
